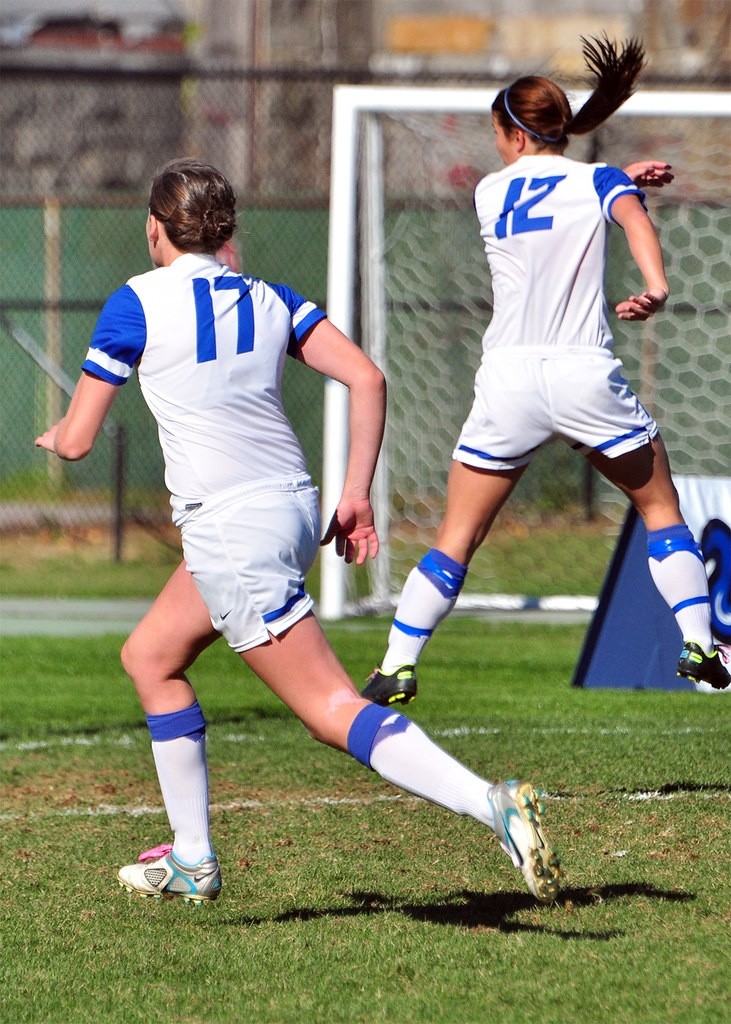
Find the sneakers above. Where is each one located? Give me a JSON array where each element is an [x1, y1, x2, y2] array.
[[676, 641, 731, 693], [486, 779, 560, 902], [116, 844, 223, 905], [359, 663, 418, 706]]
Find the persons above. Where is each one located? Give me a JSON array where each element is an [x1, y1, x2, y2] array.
[[35, 156, 561, 907], [360, 29, 731, 708]]
